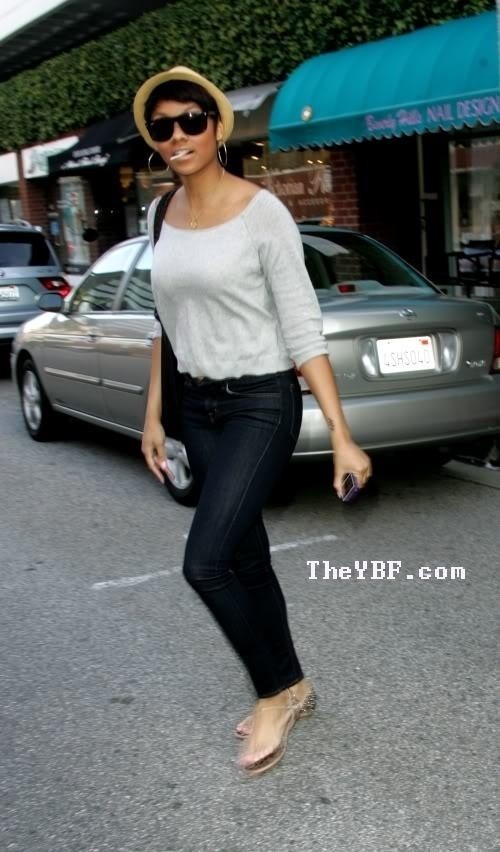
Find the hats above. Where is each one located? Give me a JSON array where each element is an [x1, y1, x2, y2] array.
[[132, 65, 234, 153]]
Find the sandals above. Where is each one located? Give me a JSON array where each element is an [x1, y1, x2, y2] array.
[[236, 689, 301, 776], [235, 680, 319, 742]]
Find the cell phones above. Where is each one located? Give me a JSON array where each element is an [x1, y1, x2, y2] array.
[[340, 471, 358, 504]]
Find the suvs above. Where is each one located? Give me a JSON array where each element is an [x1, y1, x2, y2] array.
[[0, 215, 74, 340]]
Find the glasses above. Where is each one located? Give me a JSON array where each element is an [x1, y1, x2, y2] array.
[[145, 111, 217, 142]]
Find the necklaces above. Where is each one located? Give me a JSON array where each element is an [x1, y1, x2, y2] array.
[[187, 168, 225, 231]]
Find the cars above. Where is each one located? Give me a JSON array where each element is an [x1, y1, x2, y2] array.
[[7, 223, 498, 514]]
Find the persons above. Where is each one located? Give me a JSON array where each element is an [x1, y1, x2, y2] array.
[[133, 63, 377, 773]]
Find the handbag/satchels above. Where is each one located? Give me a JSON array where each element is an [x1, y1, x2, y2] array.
[[142, 181, 201, 507]]
